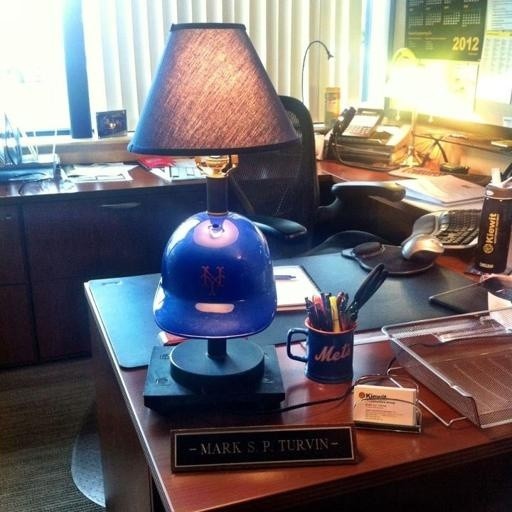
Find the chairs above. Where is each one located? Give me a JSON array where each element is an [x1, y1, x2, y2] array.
[[224, 91, 407, 259]]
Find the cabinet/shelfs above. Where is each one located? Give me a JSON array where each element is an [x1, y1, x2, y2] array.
[[0, 178, 39, 373]]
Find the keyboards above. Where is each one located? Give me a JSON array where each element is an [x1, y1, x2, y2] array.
[[400, 209, 482, 250]]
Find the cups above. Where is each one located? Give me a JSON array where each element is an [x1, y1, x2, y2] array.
[[474, 186, 512, 274], [284, 317, 358, 385]]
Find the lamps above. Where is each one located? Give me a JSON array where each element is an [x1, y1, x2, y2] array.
[[127, 22, 295, 414], [301, 39, 335, 104]]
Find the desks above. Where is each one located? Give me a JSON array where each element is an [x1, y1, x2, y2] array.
[[19, 146, 337, 366], [79, 252, 512, 512], [315, 156, 489, 245]]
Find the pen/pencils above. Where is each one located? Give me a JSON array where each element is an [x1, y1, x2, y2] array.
[[304, 292, 358, 333]]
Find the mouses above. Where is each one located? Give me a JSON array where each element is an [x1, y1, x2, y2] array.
[[402, 234, 444, 260]]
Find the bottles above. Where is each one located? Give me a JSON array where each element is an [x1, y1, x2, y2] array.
[[325, 88, 340, 127]]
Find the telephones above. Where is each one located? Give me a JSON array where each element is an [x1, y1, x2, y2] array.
[[332, 106, 384, 138]]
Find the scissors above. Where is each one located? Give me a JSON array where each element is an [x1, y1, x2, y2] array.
[[345, 263, 389, 314]]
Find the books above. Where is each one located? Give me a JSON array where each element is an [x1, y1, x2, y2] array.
[[273, 265, 322, 312], [396, 174, 487, 207]]
